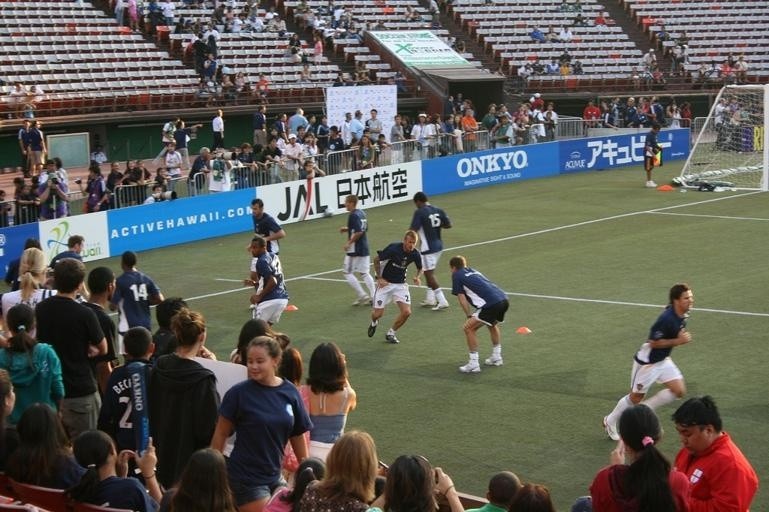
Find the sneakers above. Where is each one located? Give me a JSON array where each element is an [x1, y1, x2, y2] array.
[[647, 181, 656, 187], [603, 416, 619, 441], [352, 294, 503, 373]]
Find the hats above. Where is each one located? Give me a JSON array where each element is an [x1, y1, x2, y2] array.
[[288, 134, 296, 139], [534, 93, 541, 98]]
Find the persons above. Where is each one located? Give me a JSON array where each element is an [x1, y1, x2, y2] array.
[[601, 279, 694, 444], [296, 431, 554, 512], [339, 193, 379, 309], [0, 236, 356, 512], [568, 493, 593, 511], [404, 190, 455, 313], [2, 0, 769, 233], [588, 402, 691, 511], [245, 196, 287, 310], [667, 393, 760, 512]]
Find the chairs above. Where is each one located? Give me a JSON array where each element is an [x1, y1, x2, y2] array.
[[1, 1, 769, 110], [1, 477, 131, 512]]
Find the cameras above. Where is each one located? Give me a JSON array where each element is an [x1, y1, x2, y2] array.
[[52, 178, 59, 184], [75, 179, 81, 184]]
